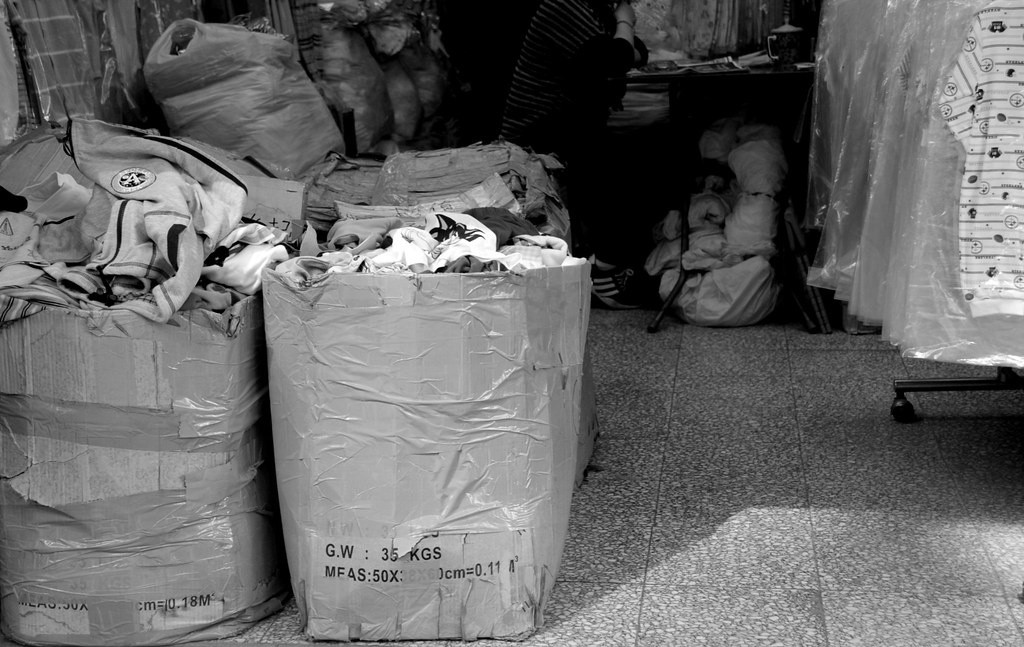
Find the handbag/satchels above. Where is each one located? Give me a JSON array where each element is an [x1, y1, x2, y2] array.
[[144, 18, 346, 180]]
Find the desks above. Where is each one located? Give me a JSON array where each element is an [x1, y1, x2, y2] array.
[[622, 61, 836, 338]]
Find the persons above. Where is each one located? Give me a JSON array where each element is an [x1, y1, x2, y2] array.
[[498, 0, 649, 310]]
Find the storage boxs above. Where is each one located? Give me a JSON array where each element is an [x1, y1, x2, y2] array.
[[0, 255, 602, 647]]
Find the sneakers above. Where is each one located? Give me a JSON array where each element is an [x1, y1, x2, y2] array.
[[588, 254, 641, 308]]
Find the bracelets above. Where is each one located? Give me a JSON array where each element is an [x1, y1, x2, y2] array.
[[616, 20, 633, 28]]
